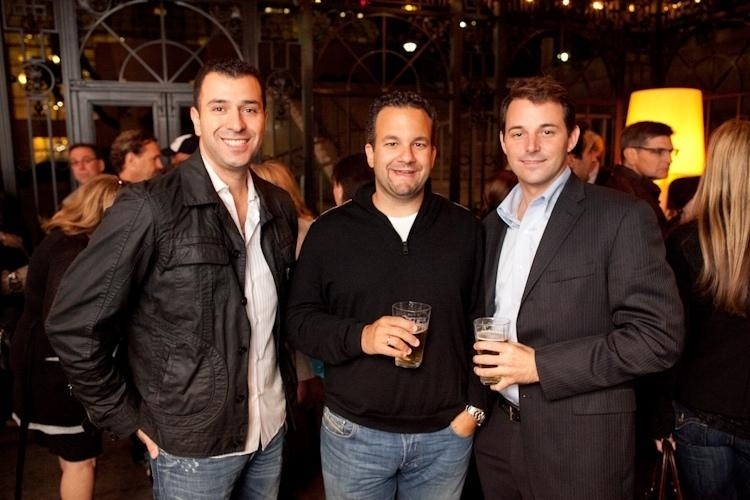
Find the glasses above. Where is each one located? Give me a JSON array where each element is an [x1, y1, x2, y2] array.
[[634, 145, 678, 156]]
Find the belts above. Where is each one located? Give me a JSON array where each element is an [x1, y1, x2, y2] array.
[[497, 396, 520, 423]]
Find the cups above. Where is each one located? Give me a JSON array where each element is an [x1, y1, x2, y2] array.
[[473, 317, 511, 386], [391, 300, 432, 368]]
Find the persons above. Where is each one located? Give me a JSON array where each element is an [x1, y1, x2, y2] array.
[[1, 56, 750, 500]]
